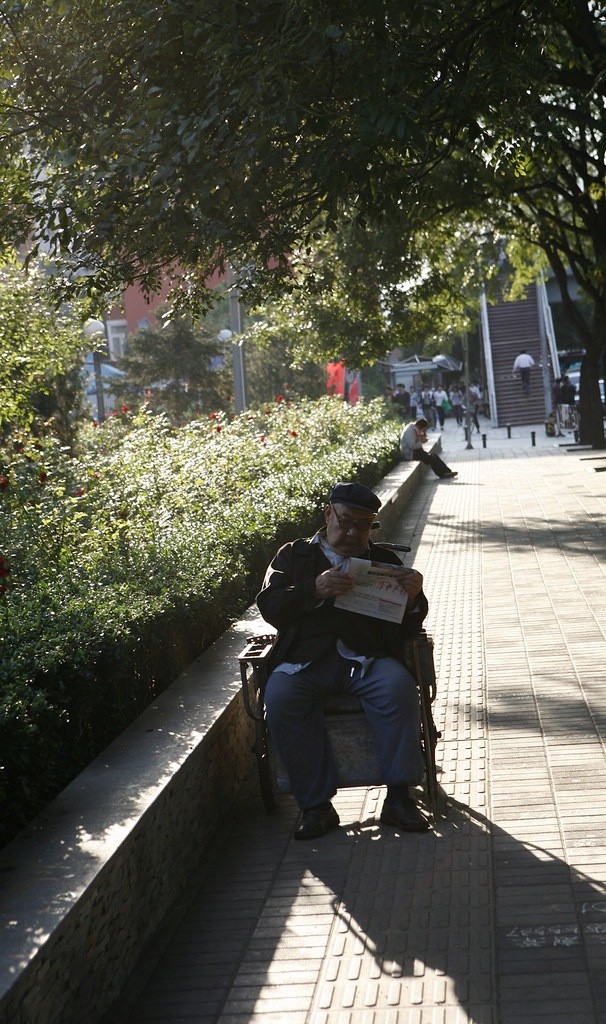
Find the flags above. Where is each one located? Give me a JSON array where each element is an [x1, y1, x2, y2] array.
[[349, 377, 358, 404], [325, 358, 344, 400]]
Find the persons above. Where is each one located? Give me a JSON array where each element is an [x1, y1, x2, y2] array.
[[256, 483, 428, 841], [551, 376, 576, 411], [384, 387, 394, 403], [410, 386, 419, 419], [399, 419, 458, 478], [512, 350, 534, 393], [393, 384, 410, 418], [418, 381, 483, 434]]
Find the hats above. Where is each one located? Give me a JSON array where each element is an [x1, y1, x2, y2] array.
[[330, 482, 382, 514]]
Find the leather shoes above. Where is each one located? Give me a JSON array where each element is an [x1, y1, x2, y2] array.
[[379, 797, 431, 833], [293, 804, 341, 841]]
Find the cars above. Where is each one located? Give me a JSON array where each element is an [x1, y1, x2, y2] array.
[[560, 369, 606, 406], [84, 363, 151, 423]]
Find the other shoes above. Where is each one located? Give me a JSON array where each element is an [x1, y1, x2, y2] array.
[[440, 472, 458, 478]]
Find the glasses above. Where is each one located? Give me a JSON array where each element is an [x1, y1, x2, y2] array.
[[332, 504, 374, 531]]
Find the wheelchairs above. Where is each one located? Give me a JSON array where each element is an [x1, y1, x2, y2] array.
[[239, 518, 442, 825]]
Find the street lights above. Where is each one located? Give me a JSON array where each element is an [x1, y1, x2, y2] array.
[[83, 317, 107, 424], [217, 329, 246, 418]]
